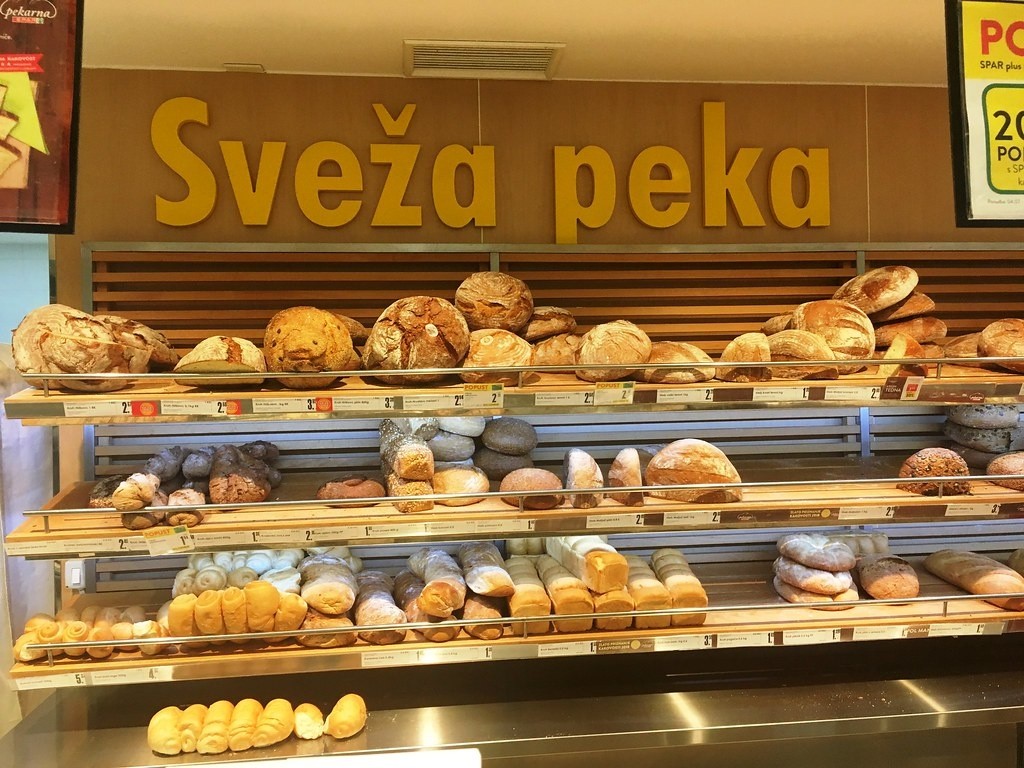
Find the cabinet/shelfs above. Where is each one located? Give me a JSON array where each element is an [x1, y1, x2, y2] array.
[[0, 352, 1024, 693]]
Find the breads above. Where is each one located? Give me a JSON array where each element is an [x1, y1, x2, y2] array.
[[11, 266, 1024, 390], [85, 401, 1024, 531], [148, 694, 367, 756], [12, 528, 1024, 661]]
